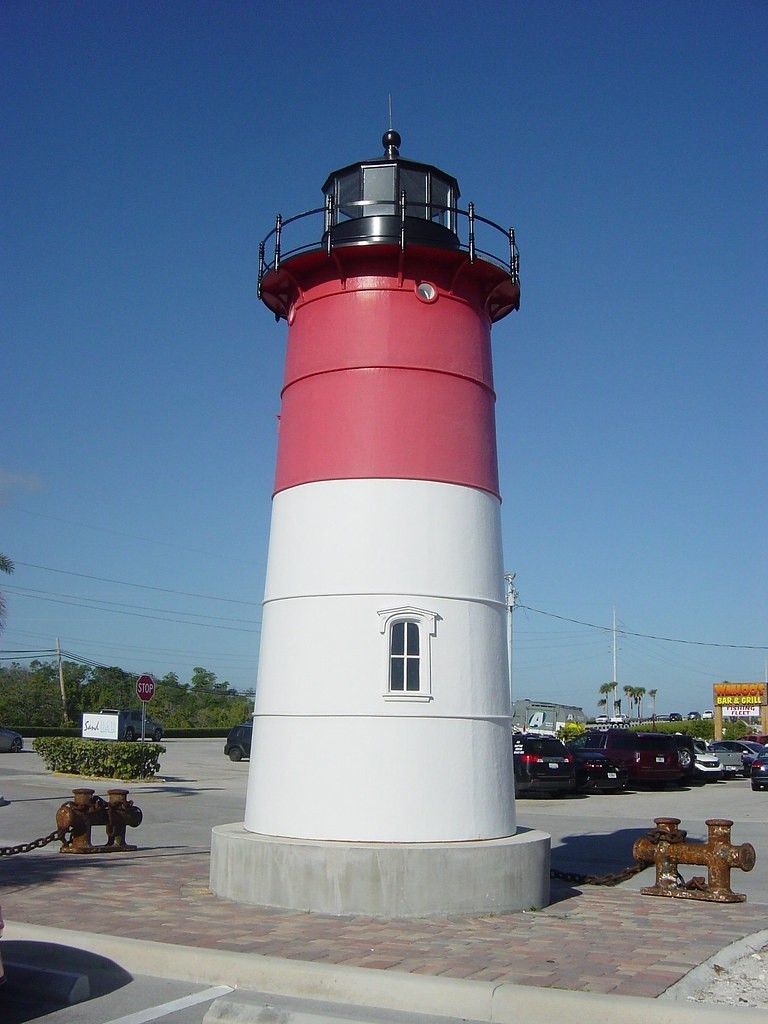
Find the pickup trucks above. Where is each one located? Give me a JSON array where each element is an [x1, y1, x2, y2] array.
[[610, 714, 630, 723]]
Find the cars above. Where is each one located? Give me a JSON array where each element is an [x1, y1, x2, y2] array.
[[702, 709, 714, 720], [688, 712, 701, 721], [691, 746, 727, 785], [668, 713, 683, 722], [0, 726, 22, 754], [744, 745, 768, 791], [648, 715, 670, 722], [595, 714, 609, 724], [734, 734, 768, 746], [700, 739, 765, 780], [566, 749, 629, 795]]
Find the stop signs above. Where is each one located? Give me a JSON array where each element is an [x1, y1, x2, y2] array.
[[135, 675, 155, 702]]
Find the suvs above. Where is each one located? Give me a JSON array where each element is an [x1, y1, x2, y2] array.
[[512, 733, 576, 800], [568, 729, 695, 791], [223, 722, 253, 761], [97, 707, 164, 742]]
